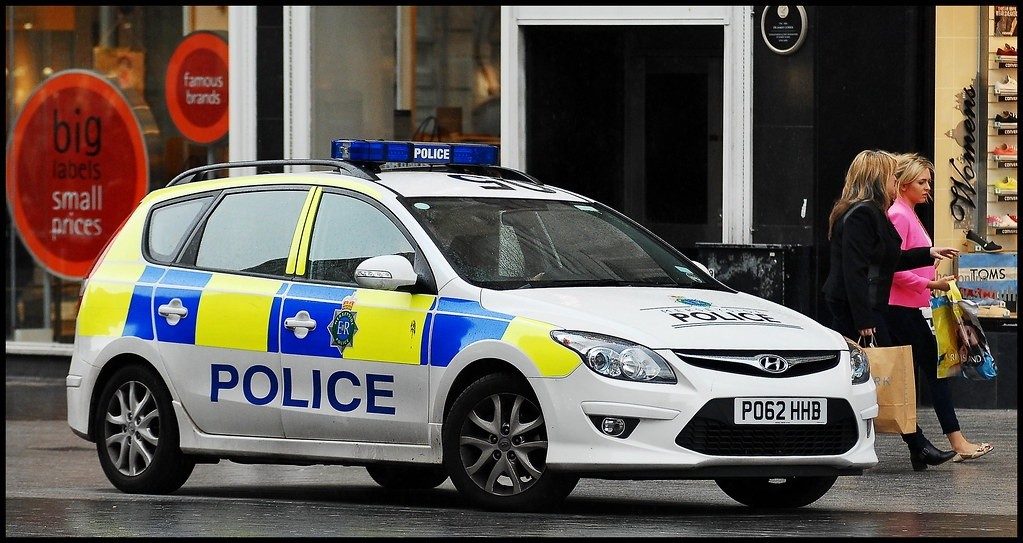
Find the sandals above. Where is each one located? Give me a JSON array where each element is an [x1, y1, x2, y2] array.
[[952, 443, 993, 462]]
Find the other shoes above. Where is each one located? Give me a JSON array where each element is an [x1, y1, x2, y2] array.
[[987, 214, 1017, 229]]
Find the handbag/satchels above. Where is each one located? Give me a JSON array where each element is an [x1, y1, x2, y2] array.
[[857, 333, 917, 434], [928, 279, 999, 382]]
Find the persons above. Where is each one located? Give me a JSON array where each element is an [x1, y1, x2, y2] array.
[[932, 304, 986, 378], [826, 149, 960, 472], [881, 153, 995, 463]]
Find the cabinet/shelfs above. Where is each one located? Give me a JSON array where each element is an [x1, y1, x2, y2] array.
[[978, 5, 1018, 248]]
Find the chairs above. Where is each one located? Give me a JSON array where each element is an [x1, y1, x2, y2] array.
[[448, 230, 542, 281]]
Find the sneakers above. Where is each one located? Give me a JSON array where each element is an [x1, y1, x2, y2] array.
[[993, 110, 1017, 129], [994, 176, 1017, 194], [994, 74, 1017, 95], [992, 143, 1017, 160], [995, 43, 1017, 62]]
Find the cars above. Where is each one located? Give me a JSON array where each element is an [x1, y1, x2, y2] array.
[[67, 139, 878, 513]]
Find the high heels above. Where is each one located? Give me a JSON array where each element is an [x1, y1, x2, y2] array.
[[910, 440, 957, 471], [966, 230, 1003, 253]]
